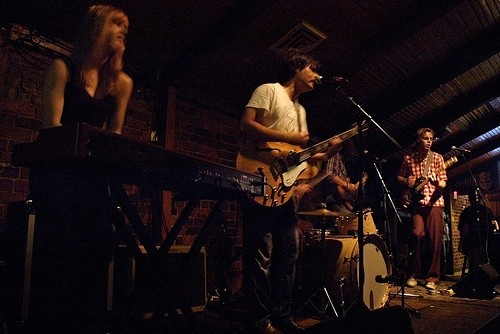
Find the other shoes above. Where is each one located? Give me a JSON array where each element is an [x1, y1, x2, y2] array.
[[406, 277, 417, 286], [426, 281, 437, 290]]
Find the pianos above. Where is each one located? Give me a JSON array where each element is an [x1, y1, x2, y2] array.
[[10, 123, 266, 334]]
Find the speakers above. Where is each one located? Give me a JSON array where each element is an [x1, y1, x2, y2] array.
[[301, 305, 415, 334], [105, 246, 206, 320]]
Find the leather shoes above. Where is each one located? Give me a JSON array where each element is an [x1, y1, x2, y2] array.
[[271, 316, 306, 334], [245, 321, 283, 334]]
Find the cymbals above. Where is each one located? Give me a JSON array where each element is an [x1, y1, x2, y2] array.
[[294, 210, 349, 216]]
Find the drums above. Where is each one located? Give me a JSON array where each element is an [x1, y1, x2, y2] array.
[[335, 208, 380, 236], [302, 234, 392, 312]]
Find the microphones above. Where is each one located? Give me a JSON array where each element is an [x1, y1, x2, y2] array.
[[375, 275, 403, 284], [451, 146, 471, 152], [362, 150, 381, 161], [315, 76, 344, 85]]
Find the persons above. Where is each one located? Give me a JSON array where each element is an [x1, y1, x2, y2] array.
[[240, 55, 320, 334], [395, 127, 447, 290], [322, 151, 358, 212], [458, 194, 496, 273], [30, 5, 134, 334]]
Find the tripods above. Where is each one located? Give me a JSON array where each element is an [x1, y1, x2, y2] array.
[[295, 218, 339, 319]]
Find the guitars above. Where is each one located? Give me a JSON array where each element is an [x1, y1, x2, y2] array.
[[402, 156, 458, 209], [337, 171, 368, 211], [236, 119, 369, 207]]
